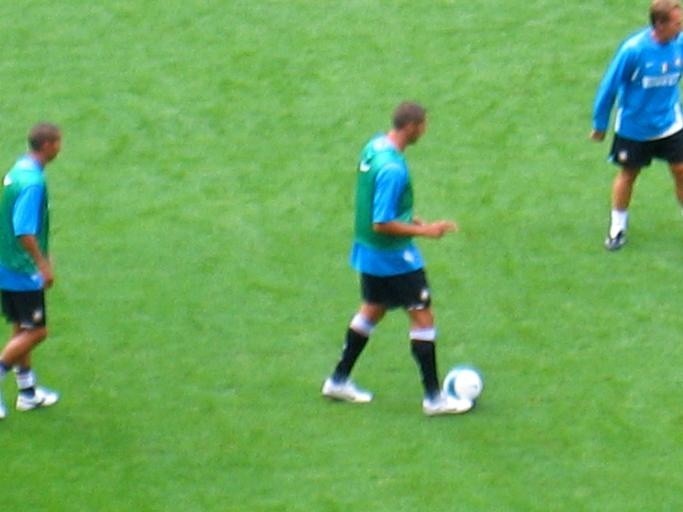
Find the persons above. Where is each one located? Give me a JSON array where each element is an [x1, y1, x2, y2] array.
[[1, 123, 64, 420], [586, 0, 682, 253], [317, 99, 473, 416]]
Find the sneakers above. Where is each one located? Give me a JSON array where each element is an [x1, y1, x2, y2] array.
[[605, 224, 627, 249], [423, 390, 474, 416], [16, 386, 59, 411], [321, 377, 373, 404]]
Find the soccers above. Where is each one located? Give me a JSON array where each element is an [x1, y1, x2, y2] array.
[[443, 368, 481, 402]]
